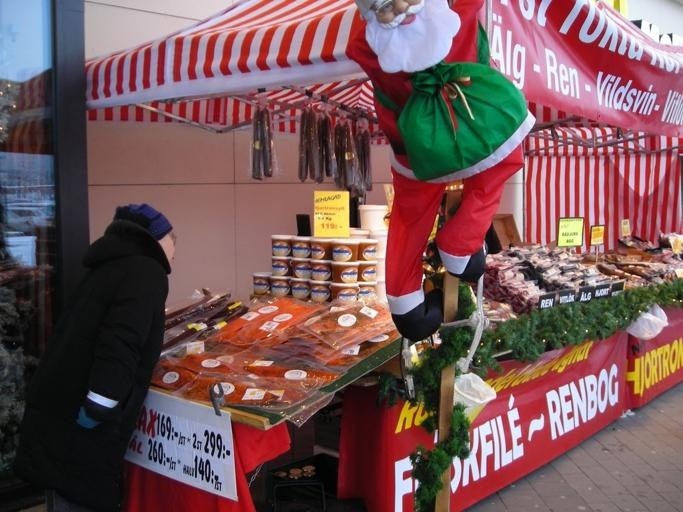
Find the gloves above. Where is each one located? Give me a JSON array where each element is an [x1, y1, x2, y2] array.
[[72, 406, 104, 432]]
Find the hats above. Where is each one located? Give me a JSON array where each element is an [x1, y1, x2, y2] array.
[[114, 202, 174, 242]]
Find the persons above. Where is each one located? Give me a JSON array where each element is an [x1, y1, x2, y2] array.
[[11, 202, 177, 512], [346, 0, 536, 343]]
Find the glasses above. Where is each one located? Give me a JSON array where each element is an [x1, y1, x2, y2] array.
[[166, 229, 177, 242]]
